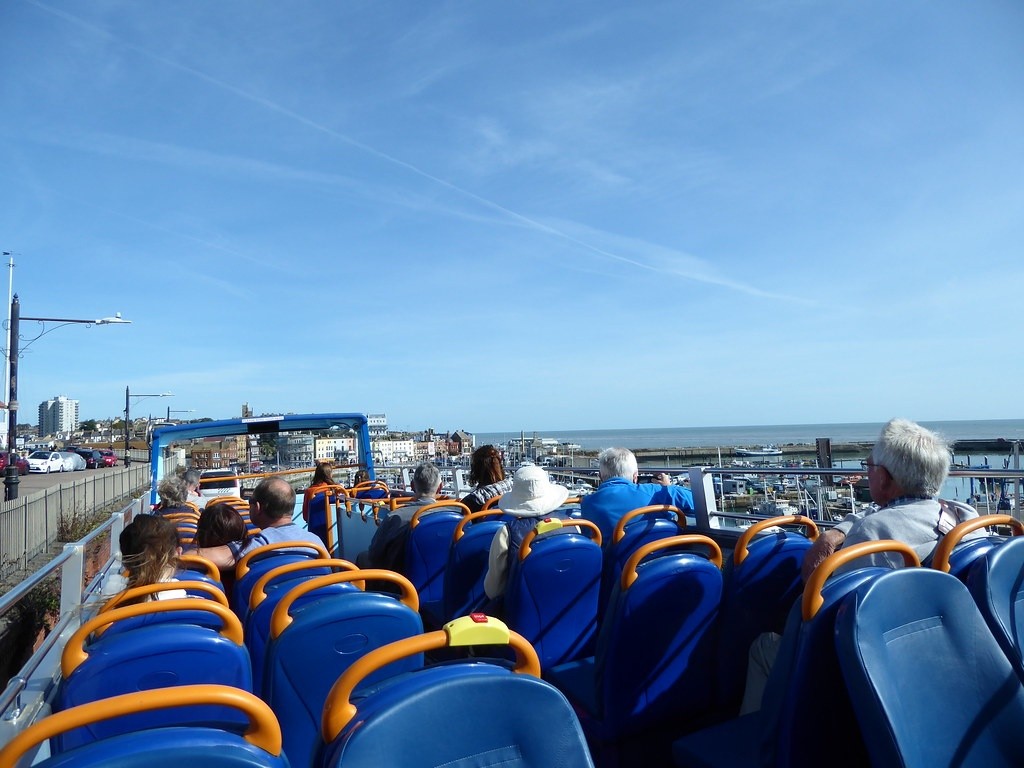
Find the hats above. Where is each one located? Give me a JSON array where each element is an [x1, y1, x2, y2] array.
[[497, 466, 569, 517]]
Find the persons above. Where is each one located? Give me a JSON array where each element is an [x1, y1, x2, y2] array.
[[181, 476, 331, 569], [303, 462, 341, 521], [191, 504, 249, 548], [460, 445, 514, 523], [484, 466, 583, 598], [368, 462, 453, 569], [354, 470, 370, 488], [582, 447, 696, 547], [185, 469, 208, 508], [741, 417, 1000, 717], [125, 449, 131, 468], [77, 514, 206, 638], [154, 476, 194, 514]]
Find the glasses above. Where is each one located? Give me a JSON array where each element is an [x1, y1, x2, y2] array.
[[860, 459, 896, 480]]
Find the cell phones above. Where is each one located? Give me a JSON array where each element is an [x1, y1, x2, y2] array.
[[636, 476, 659, 484]]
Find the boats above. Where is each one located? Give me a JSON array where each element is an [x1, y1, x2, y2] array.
[[735, 447, 783, 457]]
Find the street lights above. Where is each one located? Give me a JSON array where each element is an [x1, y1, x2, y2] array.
[[121, 385, 175, 468], [0, 248, 14, 452], [2, 290, 133, 503], [166, 406, 197, 423]]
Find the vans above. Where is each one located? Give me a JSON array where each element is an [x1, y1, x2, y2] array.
[[196, 467, 241, 503]]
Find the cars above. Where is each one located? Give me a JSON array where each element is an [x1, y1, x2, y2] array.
[[76, 448, 106, 469], [57, 452, 87, 472], [98, 449, 118, 467], [25, 451, 63, 474], [0, 452, 30, 476], [663, 458, 847, 496]]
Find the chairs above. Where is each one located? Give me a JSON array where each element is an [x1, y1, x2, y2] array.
[[0, 479, 1024, 768]]
